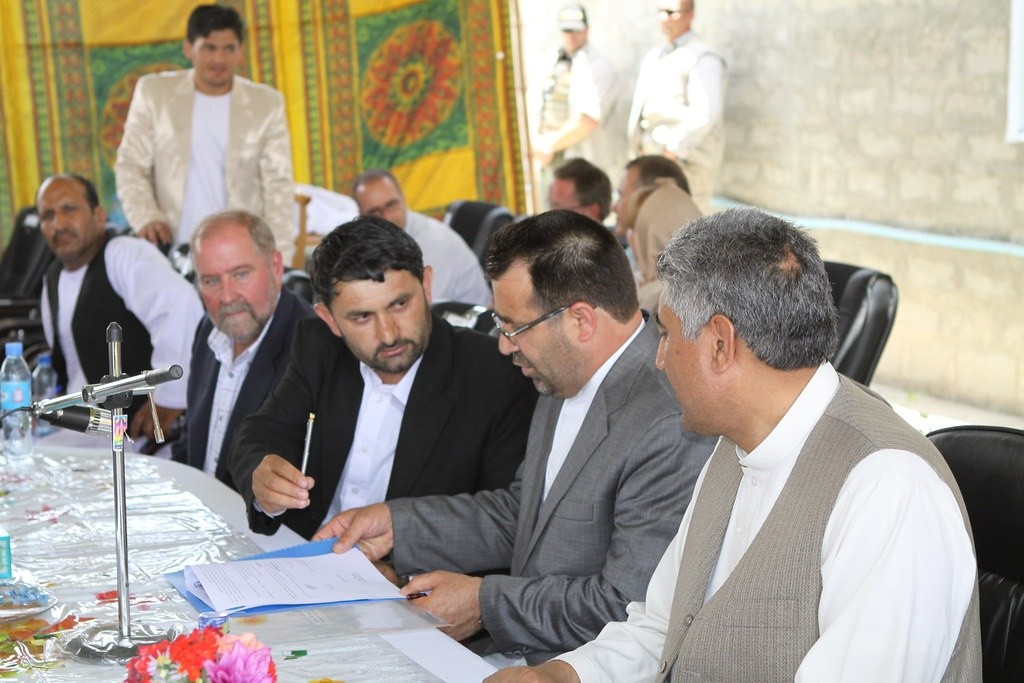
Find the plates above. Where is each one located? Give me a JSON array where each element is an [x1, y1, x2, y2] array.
[[0, 576, 60, 624]]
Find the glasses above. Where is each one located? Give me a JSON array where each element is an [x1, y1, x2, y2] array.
[[491, 301, 598, 347], [656, 8, 689, 21], [366, 199, 401, 219]]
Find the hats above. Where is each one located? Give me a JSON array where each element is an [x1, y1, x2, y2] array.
[[557, 3, 589, 32]]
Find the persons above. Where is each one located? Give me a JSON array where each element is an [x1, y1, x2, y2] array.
[[610, 155, 704, 321], [527, 1, 618, 214], [627, 0, 727, 216], [479, 207, 983, 682], [170, 170, 542, 589], [115, 4, 297, 284], [547, 158, 612, 225], [310, 209, 721, 665], [37, 172, 206, 451]]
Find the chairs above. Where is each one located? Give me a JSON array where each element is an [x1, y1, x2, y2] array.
[[443, 199, 515, 267], [0, 206, 134, 364], [921, 424, 1024, 683], [814, 259, 900, 388]]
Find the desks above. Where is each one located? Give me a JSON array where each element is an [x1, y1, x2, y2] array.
[[0, 418, 510, 683]]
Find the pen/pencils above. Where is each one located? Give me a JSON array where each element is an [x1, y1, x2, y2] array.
[[301, 411, 318, 474]]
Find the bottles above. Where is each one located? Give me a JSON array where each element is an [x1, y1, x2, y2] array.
[[0, 342, 31, 457], [31, 355, 61, 435]]
